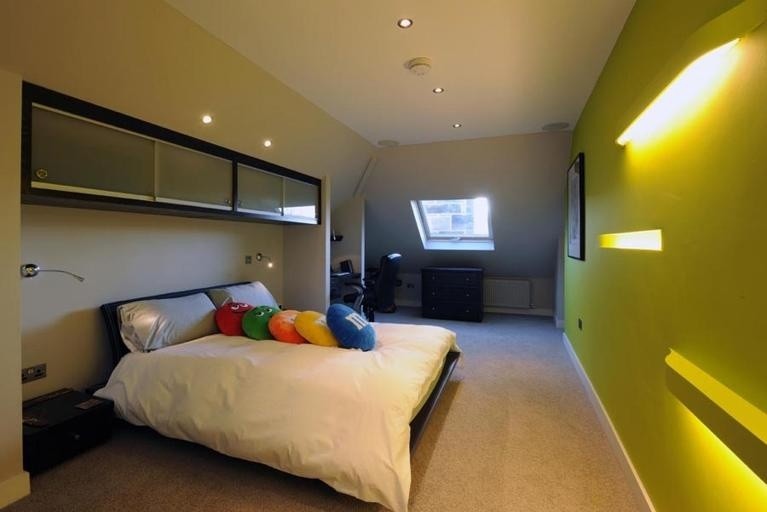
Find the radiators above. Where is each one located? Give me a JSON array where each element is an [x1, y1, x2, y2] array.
[[483, 278, 531, 309]]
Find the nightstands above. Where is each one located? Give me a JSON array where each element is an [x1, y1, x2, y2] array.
[[22, 387, 116, 476]]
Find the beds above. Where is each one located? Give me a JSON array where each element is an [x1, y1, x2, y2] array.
[[99, 282, 462, 512]]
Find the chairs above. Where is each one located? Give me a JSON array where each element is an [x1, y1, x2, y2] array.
[[343, 253, 402, 321]]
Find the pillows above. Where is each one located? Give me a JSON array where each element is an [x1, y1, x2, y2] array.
[[116, 283, 281, 352], [215, 301, 374, 351]]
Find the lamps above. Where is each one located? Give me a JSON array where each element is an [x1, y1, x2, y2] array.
[[21, 264, 85, 281], [257, 252, 273, 268]]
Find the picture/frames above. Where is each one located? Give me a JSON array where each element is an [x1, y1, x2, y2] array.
[[567, 152, 585, 261]]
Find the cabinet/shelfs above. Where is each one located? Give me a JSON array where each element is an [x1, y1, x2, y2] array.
[[23, 81, 322, 225], [421, 266, 484, 322]]
